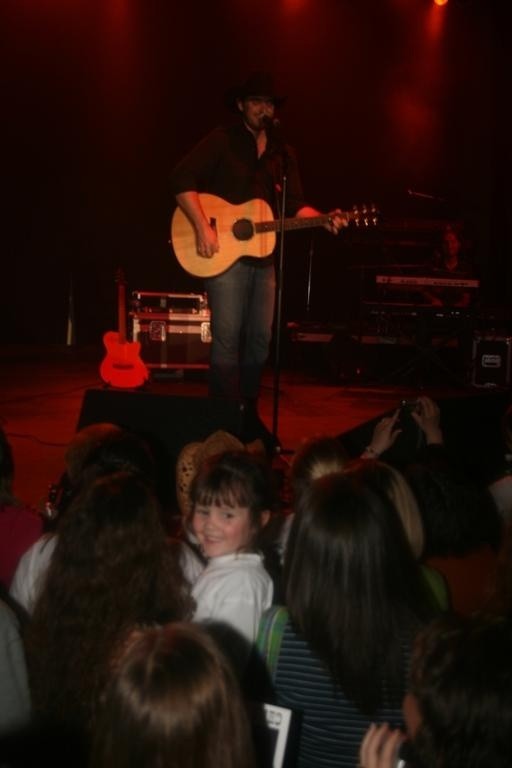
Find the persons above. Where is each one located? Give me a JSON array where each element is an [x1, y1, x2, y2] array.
[[419, 233, 476, 311], [172, 75, 348, 432], [0, 394, 511, 768]]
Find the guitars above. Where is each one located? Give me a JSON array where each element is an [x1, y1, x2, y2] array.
[[99, 265, 150, 390], [168, 192, 380, 279]]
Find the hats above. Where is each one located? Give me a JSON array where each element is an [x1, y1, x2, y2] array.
[[220, 74, 288, 116], [177, 431, 247, 517]]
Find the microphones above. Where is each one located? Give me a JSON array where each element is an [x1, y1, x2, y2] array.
[[261, 113, 281, 129]]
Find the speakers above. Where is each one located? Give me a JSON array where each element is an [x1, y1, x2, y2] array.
[[75, 390, 278, 457]]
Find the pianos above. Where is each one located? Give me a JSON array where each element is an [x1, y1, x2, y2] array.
[[373, 269, 480, 289]]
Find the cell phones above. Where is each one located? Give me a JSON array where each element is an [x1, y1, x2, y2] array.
[[251, 701, 293, 768], [399, 402, 419, 419]]
